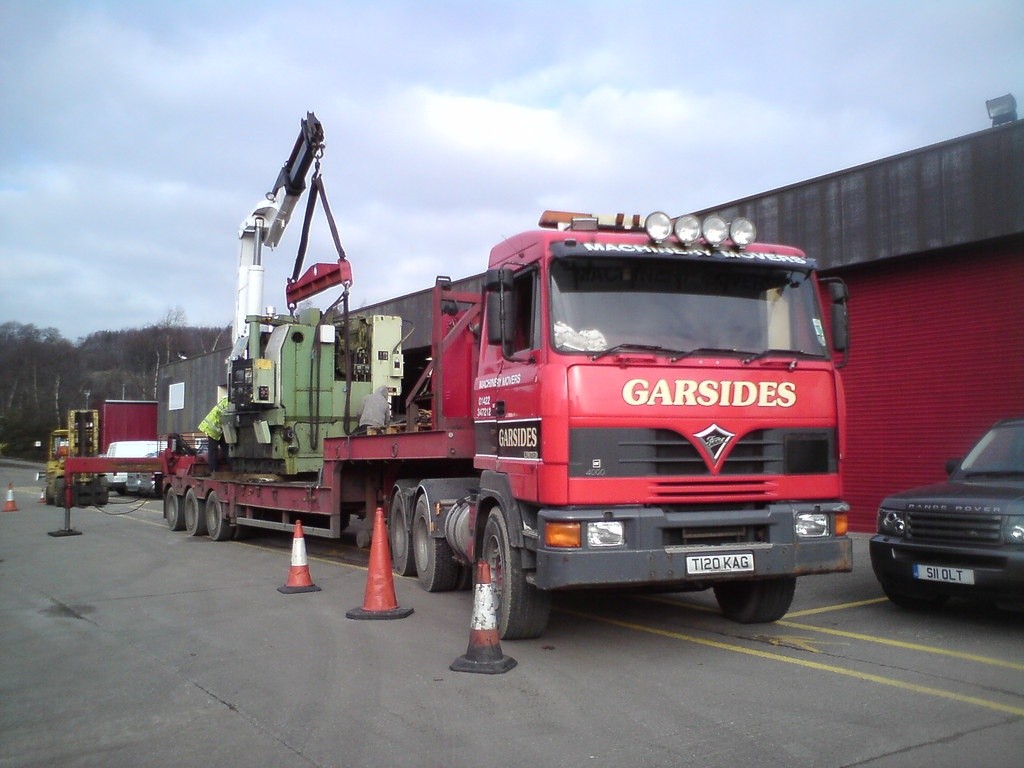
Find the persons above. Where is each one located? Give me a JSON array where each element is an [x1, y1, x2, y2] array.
[[349, 385, 389, 435], [198, 396, 229, 472]]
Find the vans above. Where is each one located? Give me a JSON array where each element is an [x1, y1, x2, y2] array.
[[99, 440, 168, 496]]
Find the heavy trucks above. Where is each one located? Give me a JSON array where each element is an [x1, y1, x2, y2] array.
[[152, 207, 856, 640]]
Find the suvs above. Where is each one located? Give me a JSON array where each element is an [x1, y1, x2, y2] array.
[[867, 414, 1024, 619], [125, 450, 163, 498]]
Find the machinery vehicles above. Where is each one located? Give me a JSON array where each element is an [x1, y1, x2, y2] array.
[[44, 406, 109, 508], [201, 107, 407, 482]]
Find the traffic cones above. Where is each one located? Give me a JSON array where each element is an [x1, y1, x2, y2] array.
[[276, 519, 322, 595], [345, 506, 415, 621], [448, 561, 519, 675], [36, 488, 47, 504], [1, 481, 19, 512]]
